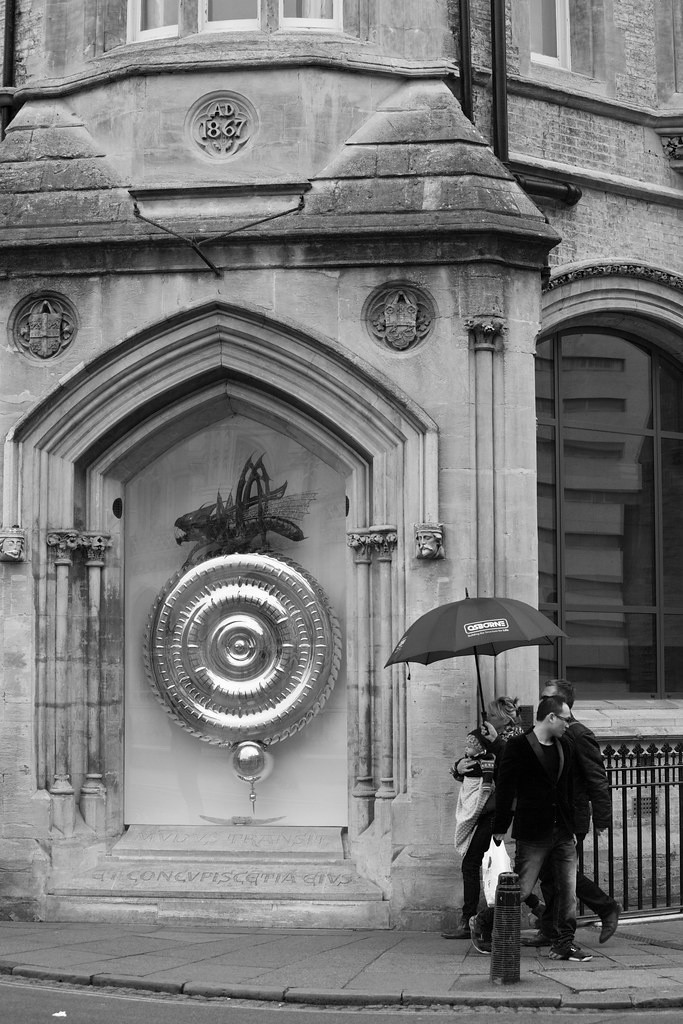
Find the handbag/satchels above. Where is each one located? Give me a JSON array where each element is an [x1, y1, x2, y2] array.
[[482, 835, 513, 908]]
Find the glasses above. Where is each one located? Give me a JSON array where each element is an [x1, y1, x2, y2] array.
[[538, 695, 552, 700], [554, 714, 572, 723]]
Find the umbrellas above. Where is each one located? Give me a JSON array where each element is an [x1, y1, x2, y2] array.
[[383, 587, 566, 733]]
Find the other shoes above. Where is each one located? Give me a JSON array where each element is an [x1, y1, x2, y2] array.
[[519, 933, 555, 948], [441, 918, 471, 938], [538, 907, 547, 928], [598, 905, 623, 944]]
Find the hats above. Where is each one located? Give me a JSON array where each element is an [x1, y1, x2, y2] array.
[[463, 728, 491, 752]]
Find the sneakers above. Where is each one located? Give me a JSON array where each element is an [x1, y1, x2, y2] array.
[[549, 941, 594, 962], [468, 911, 491, 954]]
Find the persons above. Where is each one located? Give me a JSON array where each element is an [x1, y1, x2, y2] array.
[[480, 680, 622, 947], [469, 695, 594, 963], [442, 698, 547, 939], [454, 729, 494, 857]]
